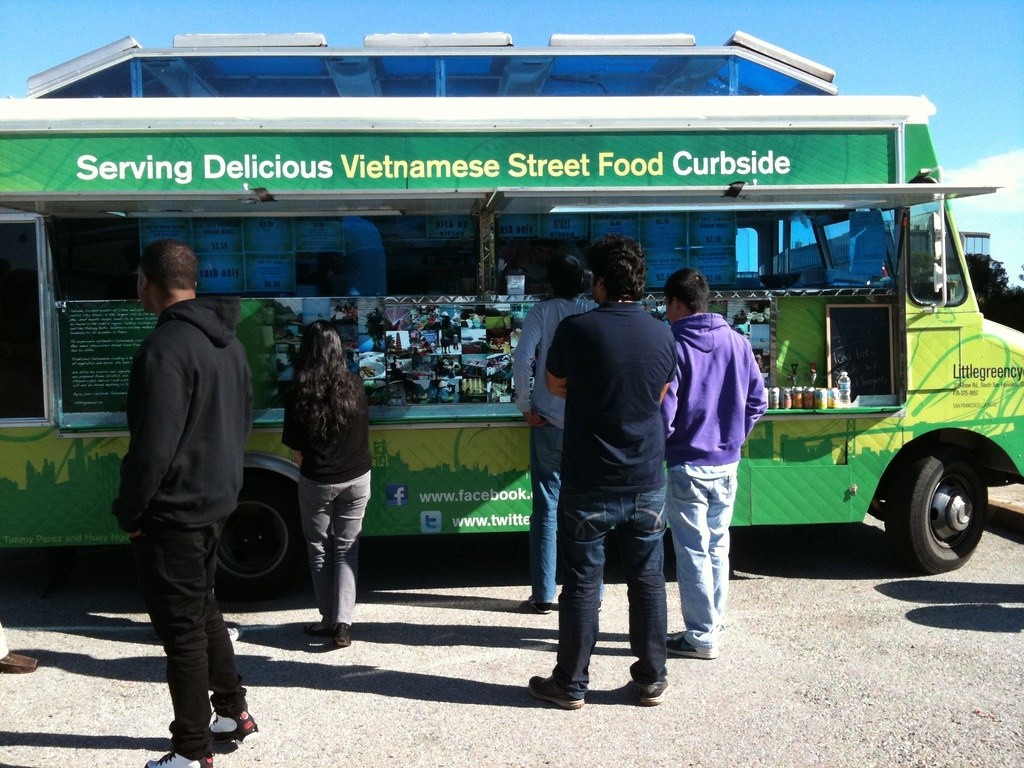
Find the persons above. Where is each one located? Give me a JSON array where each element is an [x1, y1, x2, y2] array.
[[330, 301, 509, 404], [111, 239, 262, 768], [512, 254, 603, 614], [529, 231, 677, 710], [282, 320, 371, 647], [666, 268, 766, 659]]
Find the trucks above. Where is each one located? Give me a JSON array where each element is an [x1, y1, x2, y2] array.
[[0, 91, 1024, 604]]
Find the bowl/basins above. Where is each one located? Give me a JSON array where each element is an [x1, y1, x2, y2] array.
[[760, 273, 801, 289]]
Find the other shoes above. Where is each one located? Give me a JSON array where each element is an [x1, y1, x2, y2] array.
[[304, 623, 334, 636], [0, 654, 39, 672], [334, 625, 351, 646]]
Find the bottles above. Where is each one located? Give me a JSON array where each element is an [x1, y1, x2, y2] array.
[[461, 378, 483, 394], [504, 267, 525, 295], [839, 372, 851, 407]]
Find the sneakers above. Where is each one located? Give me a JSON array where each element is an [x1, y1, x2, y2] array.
[[145, 751, 213, 768], [209, 711, 259, 744], [639, 678, 668, 704], [529, 596, 550, 613], [530, 676, 584, 708], [665, 634, 719, 658]]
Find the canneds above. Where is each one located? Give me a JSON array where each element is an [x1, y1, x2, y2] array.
[[764, 386, 840, 410]]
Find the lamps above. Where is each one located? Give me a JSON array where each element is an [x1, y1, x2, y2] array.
[[245, 187, 277, 205], [472, 189, 500, 216], [907, 167, 939, 183], [723, 180, 757, 200]]
